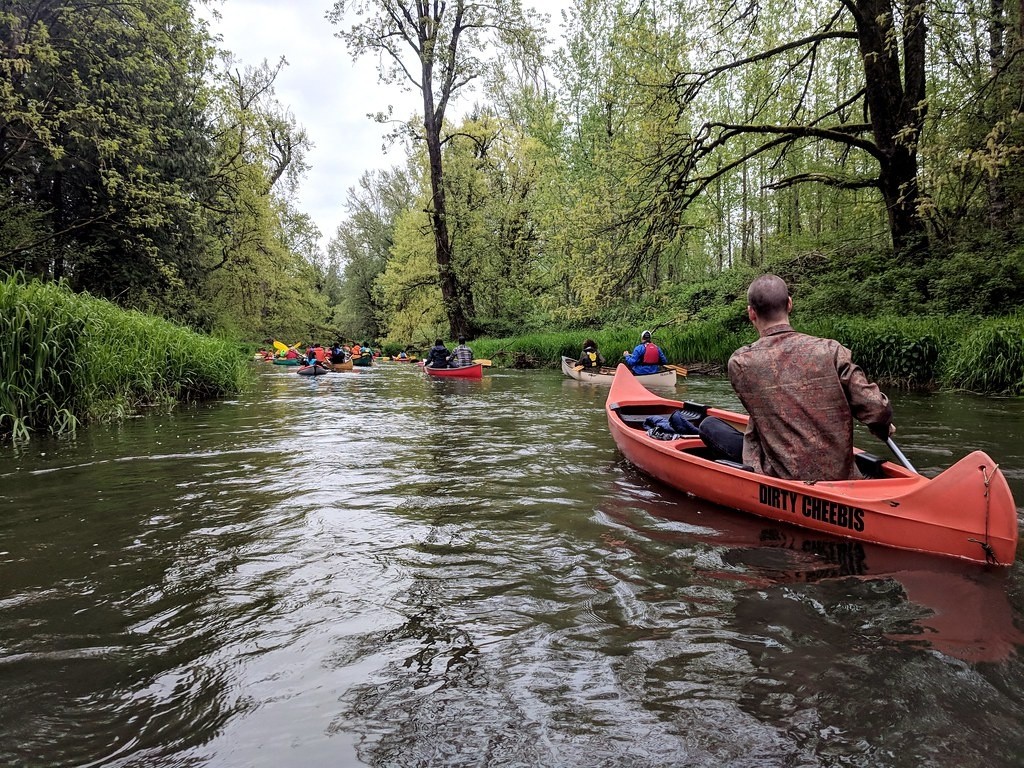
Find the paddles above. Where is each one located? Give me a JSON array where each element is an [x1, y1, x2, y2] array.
[[454, 358, 492, 367], [273, 340, 307, 358], [293, 341, 302, 348], [887, 436, 919, 474], [571, 365, 585, 371], [630, 353, 687, 377], [409, 356, 416, 361], [373, 356, 390, 361]]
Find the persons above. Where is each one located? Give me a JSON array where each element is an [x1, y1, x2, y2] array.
[[423, 338, 451, 368], [575, 339, 605, 371], [447, 336, 475, 368], [622, 330, 669, 376], [259, 341, 408, 369], [698, 274, 898, 481]]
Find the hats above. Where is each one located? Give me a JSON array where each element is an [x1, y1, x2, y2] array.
[[642, 330, 650, 339]]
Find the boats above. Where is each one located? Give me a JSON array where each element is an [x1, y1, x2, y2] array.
[[605, 362, 1017, 567], [562, 355, 677, 388], [254, 353, 373, 374], [423, 359, 483, 380], [373, 356, 424, 366]]
